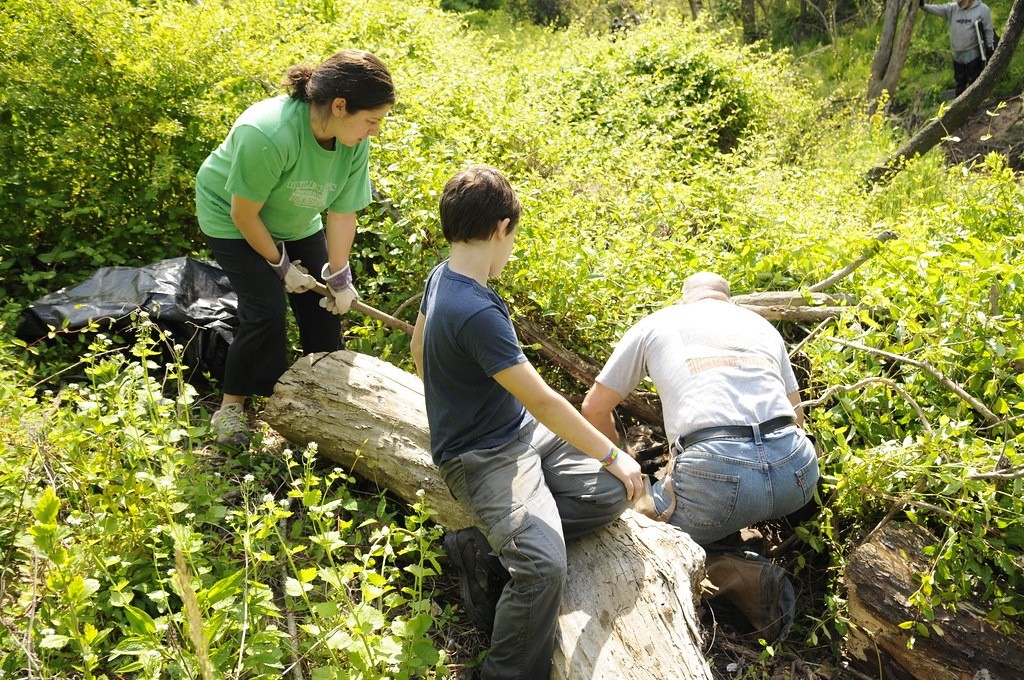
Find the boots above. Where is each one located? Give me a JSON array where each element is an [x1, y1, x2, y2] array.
[[701, 552, 795, 645]]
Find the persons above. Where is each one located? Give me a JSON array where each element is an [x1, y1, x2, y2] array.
[[410, 164, 644, 680], [581, 273, 820, 642], [194, 48, 394, 449], [918, 0, 1000, 98]]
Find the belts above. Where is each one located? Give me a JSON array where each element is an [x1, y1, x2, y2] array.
[[678, 416, 794, 454]]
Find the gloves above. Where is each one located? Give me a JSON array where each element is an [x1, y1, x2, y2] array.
[[266, 241, 317, 294], [319, 261, 359, 315]]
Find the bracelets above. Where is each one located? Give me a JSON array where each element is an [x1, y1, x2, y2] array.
[[600, 443, 618, 468]]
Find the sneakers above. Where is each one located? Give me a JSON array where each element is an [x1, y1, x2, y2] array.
[[210, 402, 250, 450], [442, 527, 499, 630]]
